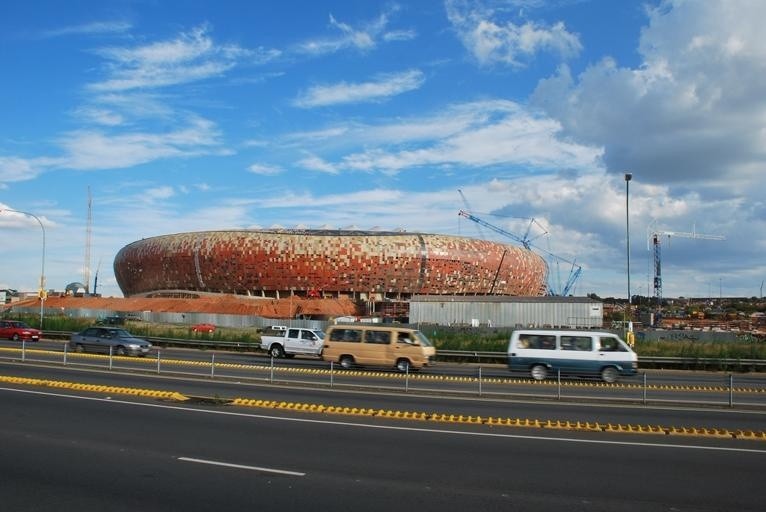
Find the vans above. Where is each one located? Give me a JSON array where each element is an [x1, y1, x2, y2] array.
[[506, 327, 639, 386], [322, 323, 439, 375]]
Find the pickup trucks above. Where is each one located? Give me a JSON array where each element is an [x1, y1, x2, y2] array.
[[259, 327, 326, 357], [255, 324, 287, 334]]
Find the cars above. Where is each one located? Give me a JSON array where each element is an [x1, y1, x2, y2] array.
[[192, 323, 215, 333], [69, 326, 153, 357], [0, 319, 42, 342]]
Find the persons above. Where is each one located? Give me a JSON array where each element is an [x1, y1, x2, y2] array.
[[329, 328, 390, 344], [518, 334, 626, 353]]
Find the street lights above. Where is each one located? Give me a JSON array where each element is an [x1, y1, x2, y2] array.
[[0, 208, 45, 330], [622, 171, 633, 317]]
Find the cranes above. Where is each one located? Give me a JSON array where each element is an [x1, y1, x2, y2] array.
[[643, 223, 729, 299]]
